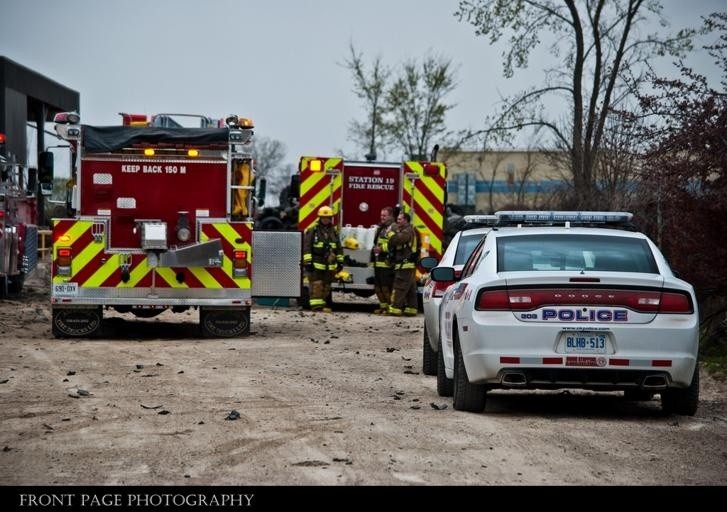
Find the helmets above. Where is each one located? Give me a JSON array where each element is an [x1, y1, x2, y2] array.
[[344, 238, 360, 250], [316, 205, 333, 217]]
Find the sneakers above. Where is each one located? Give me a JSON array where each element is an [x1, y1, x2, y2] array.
[[319, 308, 331, 313], [403, 312, 416, 316], [374, 308, 395, 315]]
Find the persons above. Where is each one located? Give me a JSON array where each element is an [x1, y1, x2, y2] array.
[[303, 206, 345, 312], [373, 207, 399, 314], [388, 213, 418, 316]]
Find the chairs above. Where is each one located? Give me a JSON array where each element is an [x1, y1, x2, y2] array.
[[596, 255, 634, 272], [501, 252, 532, 271]]
